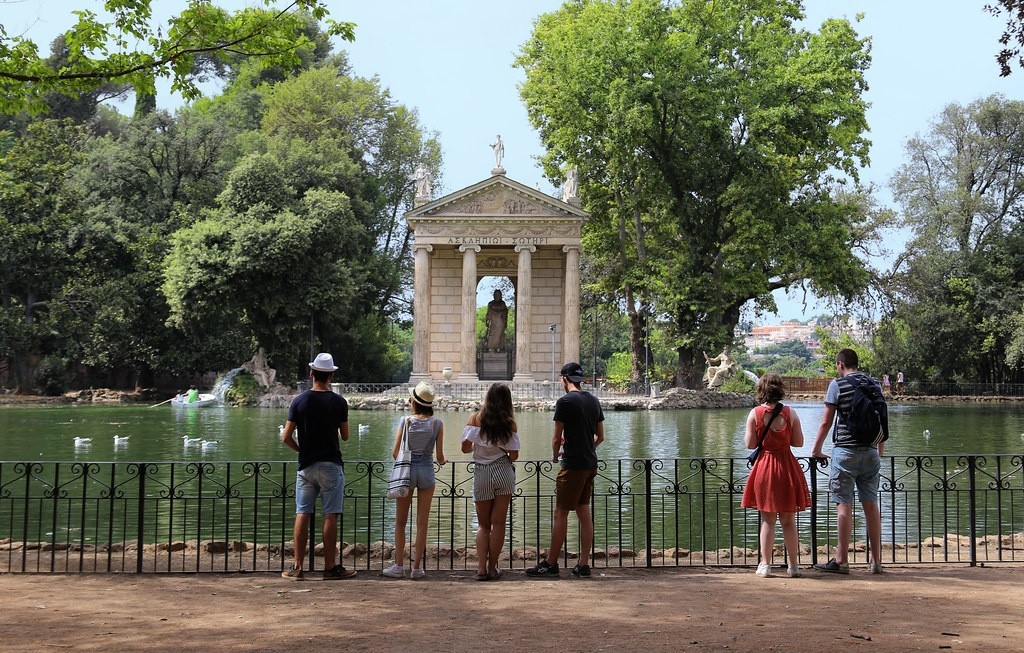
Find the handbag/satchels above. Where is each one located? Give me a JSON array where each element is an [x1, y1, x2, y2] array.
[[747, 446, 760, 466], [386, 450, 412, 499]]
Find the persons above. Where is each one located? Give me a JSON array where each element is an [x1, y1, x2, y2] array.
[[415, 163, 431, 196], [565, 165, 578, 197], [187, 386, 201, 403], [741, 374, 812, 577], [486, 290, 508, 352], [812, 349, 885, 573], [884, 369, 903, 396], [382, 381, 449, 579], [707, 349, 738, 388], [282, 353, 358, 580], [244, 348, 276, 388], [492, 135, 504, 169], [461, 383, 521, 580], [175, 390, 183, 403], [526, 362, 605, 577]]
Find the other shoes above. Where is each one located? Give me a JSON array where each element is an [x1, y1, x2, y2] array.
[[383, 564, 405, 577], [410, 569, 425, 579], [787, 564, 801, 577], [755, 562, 771, 577]]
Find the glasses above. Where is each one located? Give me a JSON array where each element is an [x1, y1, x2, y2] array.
[[409, 398, 414, 404]]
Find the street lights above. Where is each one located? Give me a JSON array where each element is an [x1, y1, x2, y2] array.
[[549, 323, 557, 400], [638, 305, 652, 397], [585, 310, 604, 388]]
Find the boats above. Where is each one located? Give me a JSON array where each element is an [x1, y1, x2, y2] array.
[[170, 393, 216, 409]]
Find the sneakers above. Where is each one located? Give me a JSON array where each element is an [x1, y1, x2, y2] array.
[[526, 558, 559, 576], [870, 560, 882, 574], [323, 564, 357, 580], [281, 565, 304, 581], [571, 564, 592, 577], [813, 558, 849, 574]]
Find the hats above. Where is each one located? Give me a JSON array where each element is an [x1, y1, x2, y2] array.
[[561, 362, 586, 383], [408, 381, 437, 407], [308, 353, 338, 372]]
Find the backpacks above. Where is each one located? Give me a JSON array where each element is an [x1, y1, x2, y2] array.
[[837, 375, 889, 445]]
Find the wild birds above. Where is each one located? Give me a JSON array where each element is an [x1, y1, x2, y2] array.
[[113, 434, 132, 442], [276, 425, 285, 433], [72, 437, 94, 444], [180, 434, 219, 447], [294, 429, 298, 438], [359, 423, 369, 430], [922, 429, 931, 436]]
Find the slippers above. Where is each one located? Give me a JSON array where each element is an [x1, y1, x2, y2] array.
[[474, 570, 488, 579], [488, 568, 502, 580]]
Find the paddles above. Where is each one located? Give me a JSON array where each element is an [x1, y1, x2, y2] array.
[[153, 394, 185, 407], [198, 395, 225, 405]]
